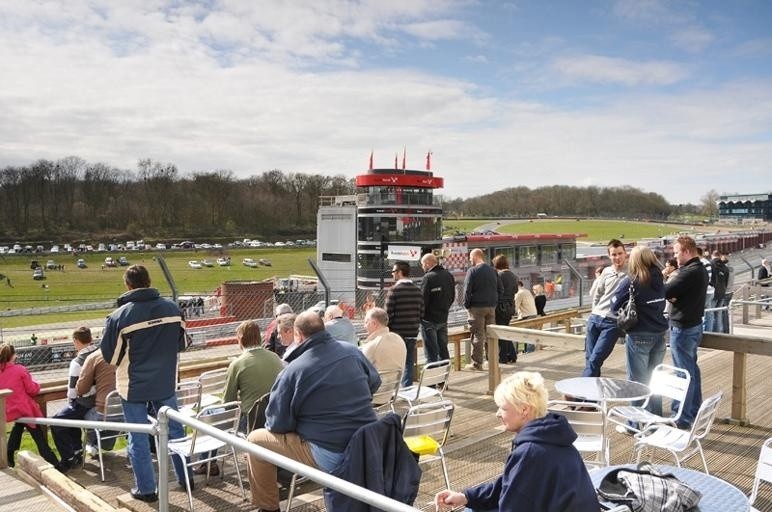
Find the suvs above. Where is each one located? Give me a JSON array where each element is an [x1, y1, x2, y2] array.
[[34, 267, 43, 280], [243, 258, 256, 267]]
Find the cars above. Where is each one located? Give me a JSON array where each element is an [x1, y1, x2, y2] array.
[[201, 259, 213, 266], [217, 258, 226, 266], [0, 238, 316, 254], [307, 300, 340, 317], [47, 260, 54, 268], [259, 259, 272, 266], [78, 258, 85, 267], [31, 260, 42, 269], [105, 257, 115, 266], [189, 260, 201, 269], [470, 229, 498, 236], [119, 256, 128, 265]]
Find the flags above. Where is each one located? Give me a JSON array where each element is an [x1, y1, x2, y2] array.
[[368, 146, 373, 169], [425, 150, 431, 170]]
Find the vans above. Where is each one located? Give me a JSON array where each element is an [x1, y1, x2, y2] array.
[[536, 213, 547, 217]]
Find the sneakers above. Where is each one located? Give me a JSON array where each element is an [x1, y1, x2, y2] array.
[[435, 357, 516, 391], [566, 394, 695, 434], [55, 444, 221, 502]]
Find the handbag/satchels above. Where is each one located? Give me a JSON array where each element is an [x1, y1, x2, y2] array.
[[618, 300, 639, 329]]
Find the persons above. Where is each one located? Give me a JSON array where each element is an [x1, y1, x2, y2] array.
[[758, 258, 771, 288], [246, 309, 382, 511], [100, 264, 193, 501], [50, 328, 119, 470], [584, 235, 709, 437], [386, 248, 455, 388], [0, 343, 59, 467], [514, 279, 554, 353], [696, 248, 729, 334], [196, 302, 407, 476], [433, 370, 602, 512], [462, 248, 517, 370]]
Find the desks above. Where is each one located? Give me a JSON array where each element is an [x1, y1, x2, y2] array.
[[581, 460, 751, 511], [553, 376, 653, 468]]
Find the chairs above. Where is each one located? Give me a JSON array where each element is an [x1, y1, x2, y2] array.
[[545, 399, 610, 469], [592, 364, 691, 463], [747, 437, 772, 512], [630, 391, 723, 477]]
